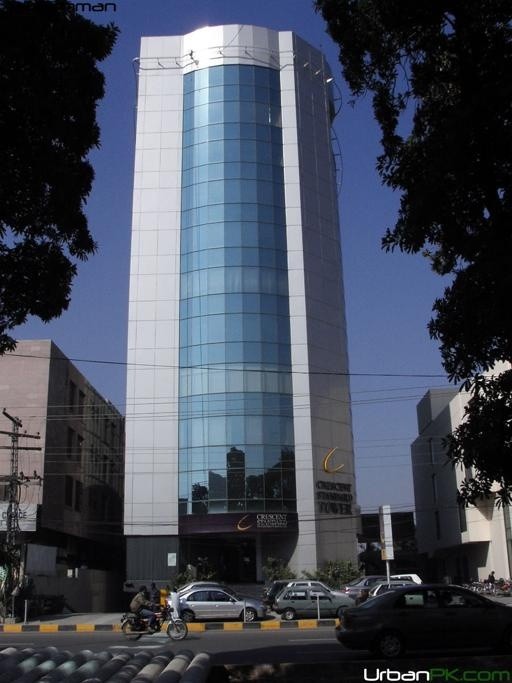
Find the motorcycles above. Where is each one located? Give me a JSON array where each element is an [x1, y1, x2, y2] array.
[[117, 600, 190, 643], [465, 576, 512, 597]]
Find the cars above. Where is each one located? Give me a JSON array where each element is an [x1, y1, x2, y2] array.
[[333, 582, 512, 661], [166, 570, 426, 622]]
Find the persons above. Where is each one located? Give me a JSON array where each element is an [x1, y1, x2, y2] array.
[[130, 586, 159, 633], [489, 571, 495, 595], [150, 583, 161, 607]]
